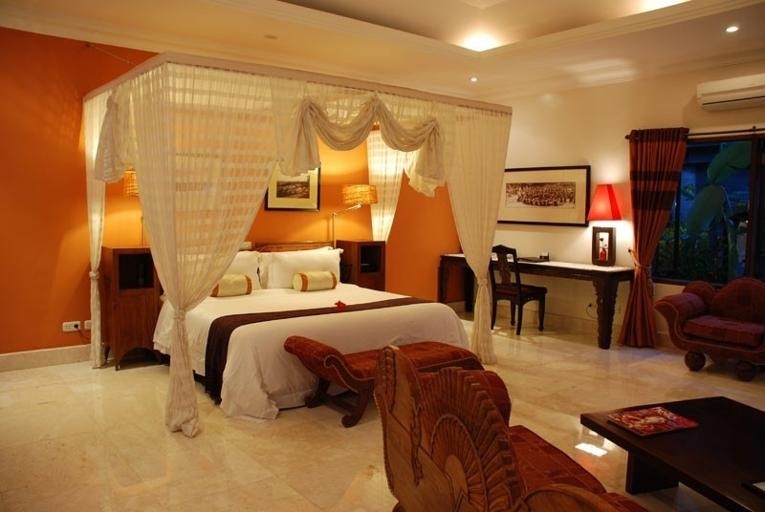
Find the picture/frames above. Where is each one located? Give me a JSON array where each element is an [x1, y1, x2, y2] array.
[[592, 226, 617, 267], [497, 165, 589, 227], [265, 159, 320, 212]]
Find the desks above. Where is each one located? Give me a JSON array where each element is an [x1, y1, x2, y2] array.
[[436, 253, 635, 350]]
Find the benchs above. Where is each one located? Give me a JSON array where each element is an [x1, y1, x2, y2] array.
[[284, 334, 486, 428]]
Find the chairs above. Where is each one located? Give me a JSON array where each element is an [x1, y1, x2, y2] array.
[[488, 245, 548, 337]]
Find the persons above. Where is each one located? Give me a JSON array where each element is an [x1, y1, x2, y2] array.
[[598, 238, 608, 261]]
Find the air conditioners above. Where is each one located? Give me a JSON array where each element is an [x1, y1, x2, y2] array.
[[696, 71, 765, 111]]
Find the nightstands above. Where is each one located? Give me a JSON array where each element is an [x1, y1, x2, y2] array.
[[333, 239, 386, 295], [100, 245, 161, 361]]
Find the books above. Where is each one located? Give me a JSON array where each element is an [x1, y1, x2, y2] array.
[[607, 406, 699, 437], [519, 257, 548, 262]]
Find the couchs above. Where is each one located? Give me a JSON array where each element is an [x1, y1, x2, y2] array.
[[653, 277, 765, 382], [372, 345, 648, 512]]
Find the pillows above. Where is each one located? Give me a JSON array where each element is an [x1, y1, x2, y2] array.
[[210, 273, 252, 297], [266, 249, 341, 289], [292, 270, 337, 291], [176, 250, 262, 293], [259, 246, 336, 286]]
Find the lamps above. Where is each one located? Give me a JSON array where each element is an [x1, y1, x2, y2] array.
[[586, 184, 622, 221], [329, 184, 378, 249]]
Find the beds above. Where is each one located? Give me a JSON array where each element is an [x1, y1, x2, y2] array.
[[157, 245, 473, 424]]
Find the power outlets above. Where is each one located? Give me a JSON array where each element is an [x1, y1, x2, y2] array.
[[83, 319, 92, 330], [62, 321, 80, 332]]
